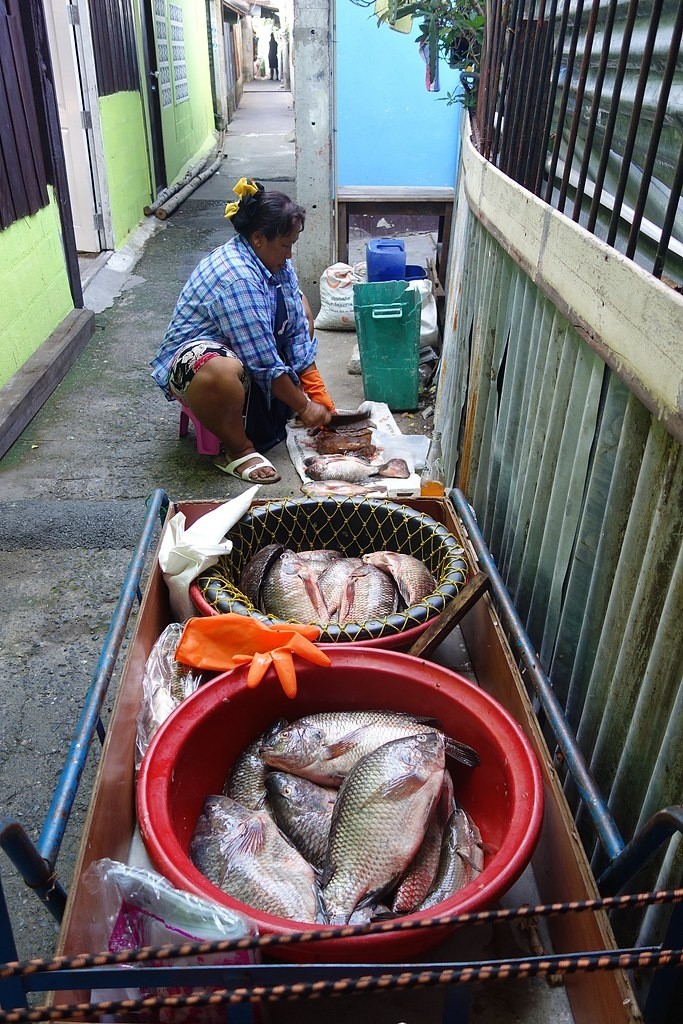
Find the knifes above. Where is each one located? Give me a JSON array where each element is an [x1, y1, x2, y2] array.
[[294, 411, 371, 432]]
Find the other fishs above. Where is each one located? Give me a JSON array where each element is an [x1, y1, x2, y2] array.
[[237, 543, 436, 626], [299, 415, 410, 496], [190, 710, 484, 926]]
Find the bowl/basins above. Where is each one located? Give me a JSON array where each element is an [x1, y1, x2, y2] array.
[[196, 493, 469, 642], [134, 647, 544, 965], [189, 574, 472, 650]]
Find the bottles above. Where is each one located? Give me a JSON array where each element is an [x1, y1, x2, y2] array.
[[418, 430, 445, 497]]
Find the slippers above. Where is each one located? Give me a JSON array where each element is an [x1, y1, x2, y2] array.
[[214, 452, 281, 484]]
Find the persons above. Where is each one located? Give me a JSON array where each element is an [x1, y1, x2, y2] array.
[[150, 176, 335, 485], [268, 33, 280, 80]]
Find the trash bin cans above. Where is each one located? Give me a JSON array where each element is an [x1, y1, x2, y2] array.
[[363, 237, 430, 282], [352, 279, 423, 412]]
